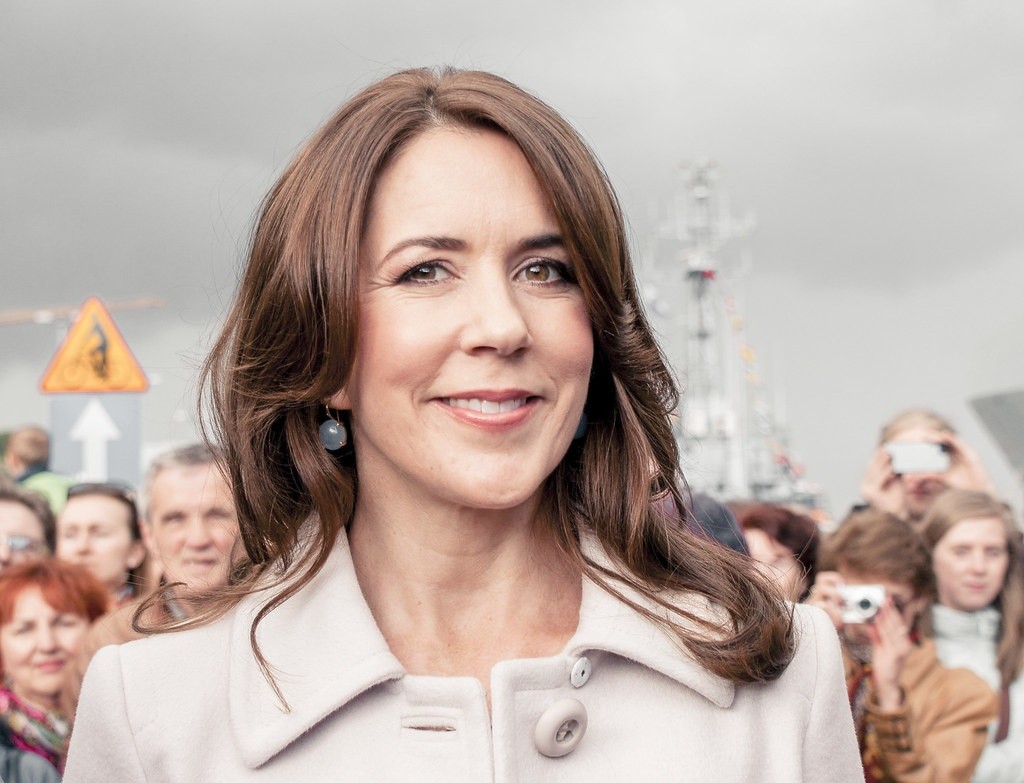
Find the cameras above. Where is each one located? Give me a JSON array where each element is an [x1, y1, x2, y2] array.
[[825, 584, 886, 624]]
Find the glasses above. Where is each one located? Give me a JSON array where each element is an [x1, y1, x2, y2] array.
[[5, 535, 39, 552], [66, 481, 138, 501]]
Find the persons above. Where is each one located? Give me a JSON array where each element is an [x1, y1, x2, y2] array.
[[62, 63, 864, 782], [0, 412, 1024, 783]]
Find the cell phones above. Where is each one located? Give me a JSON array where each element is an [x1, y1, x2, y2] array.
[[884, 442, 950, 474]]
